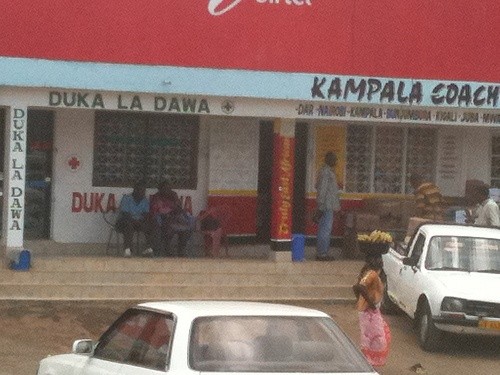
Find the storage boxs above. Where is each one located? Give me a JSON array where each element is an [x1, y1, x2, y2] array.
[[341, 199, 407, 259]]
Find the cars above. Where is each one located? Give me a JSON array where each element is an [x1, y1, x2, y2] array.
[[35, 298, 380, 372]]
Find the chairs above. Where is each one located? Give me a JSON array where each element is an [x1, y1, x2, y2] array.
[[103, 207, 142, 255], [191, 205, 233, 258]]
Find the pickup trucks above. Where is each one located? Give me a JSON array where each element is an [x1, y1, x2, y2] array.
[[380, 220, 499, 353]]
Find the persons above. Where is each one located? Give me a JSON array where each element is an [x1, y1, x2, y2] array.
[[116, 179, 153, 256], [150, 181, 191, 257], [356, 254, 391, 367], [314, 152, 340, 261], [200, 209, 228, 258], [465, 183, 500, 228]]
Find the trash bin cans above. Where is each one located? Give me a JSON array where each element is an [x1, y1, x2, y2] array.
[[292, 233, 305, 261]]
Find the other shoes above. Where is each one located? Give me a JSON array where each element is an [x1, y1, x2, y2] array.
[[124, 244, 188, 258], [315, 253, 334, 261]]
